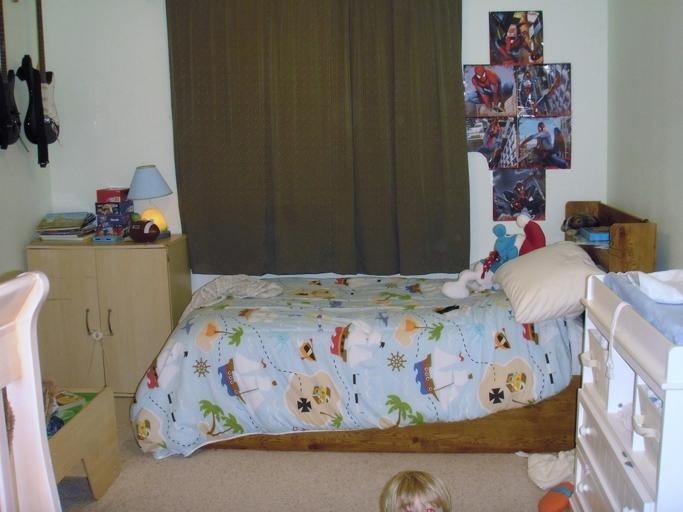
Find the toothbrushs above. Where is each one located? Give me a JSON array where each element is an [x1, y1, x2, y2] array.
[[129, 220, 159, 243]]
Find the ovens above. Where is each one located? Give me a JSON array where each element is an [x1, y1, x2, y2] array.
[[174, 201, 655, 454]]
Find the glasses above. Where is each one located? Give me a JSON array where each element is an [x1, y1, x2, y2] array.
[[127, 165, 174, 234]]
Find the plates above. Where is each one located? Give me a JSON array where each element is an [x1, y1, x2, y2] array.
[[567, 266, 683, 511], [25, 234, 195, 400]]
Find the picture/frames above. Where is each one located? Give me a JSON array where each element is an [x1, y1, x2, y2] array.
[[43, 384, 122, 501]]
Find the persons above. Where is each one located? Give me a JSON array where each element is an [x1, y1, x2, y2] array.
[[377, 469, 451, 512], [468, 17, 566, 212]]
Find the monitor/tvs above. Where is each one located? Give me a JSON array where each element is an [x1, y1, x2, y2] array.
[[56, 390, 85, 411], [34, 208, 95, 242]]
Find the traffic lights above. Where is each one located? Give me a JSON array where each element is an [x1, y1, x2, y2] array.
[[491, 240, 609, 327]]
